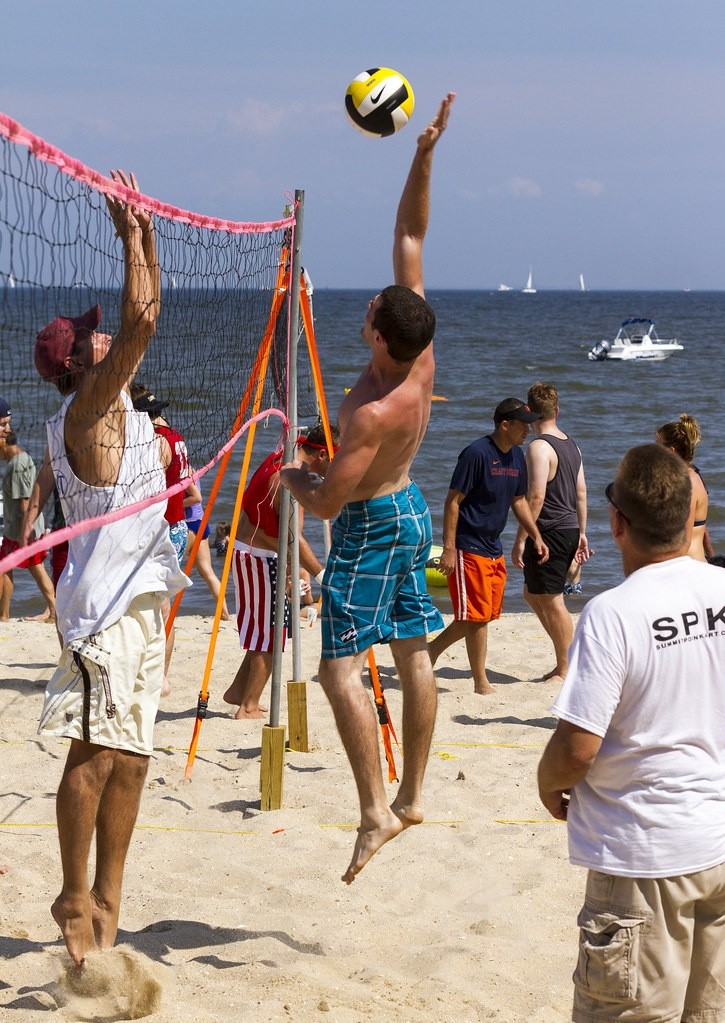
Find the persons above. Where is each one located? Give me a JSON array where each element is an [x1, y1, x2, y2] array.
[[565, 549, 595, 595], [286, 551, 321, 618], [709, 555, 725, 568], [209, 521, 231, 553], [655, 414, 714, 563], [0, 397, 69, 625], [426, 398, 550, 695], [511, 382, 590, 682], [128, 384, 187, 696], [280, 92, 456, 885], [184, 463, 232, 621], [539, 444, 725, 1023], [223, 424, 341, 720], [35, 168, 193, 966]]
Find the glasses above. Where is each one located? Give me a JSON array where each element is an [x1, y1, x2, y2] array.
[[605, 482, 631, 526]]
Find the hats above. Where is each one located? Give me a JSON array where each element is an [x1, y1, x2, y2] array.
[[0, 397, 11, 417], [493, 404, 544, 423], [133, 394, 171, 411], [34, 304, 101, 385]]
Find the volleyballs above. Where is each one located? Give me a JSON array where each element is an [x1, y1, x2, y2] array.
[[344, 67, 416, 140]]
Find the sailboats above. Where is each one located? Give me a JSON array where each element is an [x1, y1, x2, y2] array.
[[521, 266, 537, 293], [579, 273, 589, 291]]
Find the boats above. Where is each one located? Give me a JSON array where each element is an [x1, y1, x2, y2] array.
[[588, 318, 684, 361], [497, 284, 513, 291], [425, 545, 448, 587]]
